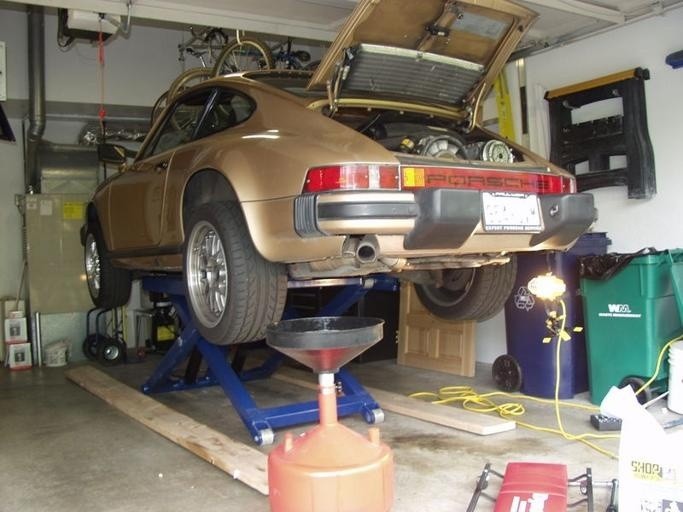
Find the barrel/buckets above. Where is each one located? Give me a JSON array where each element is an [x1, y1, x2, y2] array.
[[667, 339, 683, 416], [44, 345, 68, 367]]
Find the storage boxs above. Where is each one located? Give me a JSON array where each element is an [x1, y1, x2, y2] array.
[[3, 316, 32, 371]]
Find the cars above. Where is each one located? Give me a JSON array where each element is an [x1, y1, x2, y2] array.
[[77, 0, 602, 348]]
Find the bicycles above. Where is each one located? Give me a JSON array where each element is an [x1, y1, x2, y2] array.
[[142, 27, 325, 145]]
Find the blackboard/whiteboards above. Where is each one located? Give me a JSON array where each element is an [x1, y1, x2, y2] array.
[[25, 192, 92, 315]]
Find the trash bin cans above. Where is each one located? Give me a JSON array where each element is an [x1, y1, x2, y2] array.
[[491, 233, 682, 406]]
